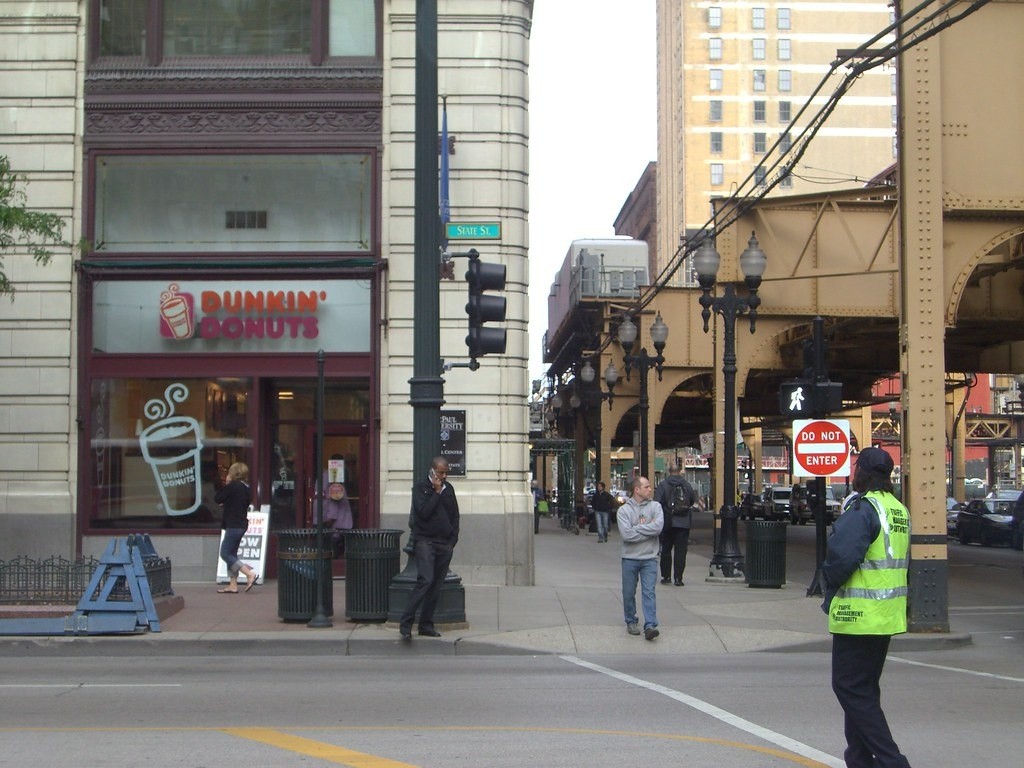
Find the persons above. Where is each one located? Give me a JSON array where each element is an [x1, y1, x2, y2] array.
[[1009, 491, 1024, 551], [546, 489, 558, 517], [531, 480, 544, 534], [617, 476, 665, 641], [399, 456, 460, 640], [653, 465, 696, 586], [590, 482, 613, 543], [820, 447, 913, 768], [215, 463, 259, 594], [737, 488, 742, 508], [789, 484, 803, 515], [313, 454, 353, 562]]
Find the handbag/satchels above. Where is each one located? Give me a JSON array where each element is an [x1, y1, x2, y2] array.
[[538, 499, 549, 512]]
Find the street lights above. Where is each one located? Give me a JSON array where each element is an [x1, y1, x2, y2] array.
[[695, 227, 768, 589], [552, 393, 581, 490], [580, 359, 619, 484], [618, 309, 670, 482]]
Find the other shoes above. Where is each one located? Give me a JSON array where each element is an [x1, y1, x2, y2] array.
[[675, 578, 685, 586], [605, 537, 607, 542], [418, 630, 441, 637], [627, 622, 641, 635], [598, 540, 603, 543], [646, 628, 660, 639], [660, 577, 671, 583], [400, 630, 412, 638]]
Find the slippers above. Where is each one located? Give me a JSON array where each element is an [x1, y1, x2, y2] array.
[[217, 588, 238, 593], [245, 574, 260, 592]]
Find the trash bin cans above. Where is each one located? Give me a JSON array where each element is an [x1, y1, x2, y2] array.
[[742, 520, 790, 587], [272, 528, 338, 619], [336, 527, 405, 621]]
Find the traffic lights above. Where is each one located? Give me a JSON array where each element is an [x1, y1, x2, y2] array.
[[780, 381, 814, 418], [463, 248, 507, 359]]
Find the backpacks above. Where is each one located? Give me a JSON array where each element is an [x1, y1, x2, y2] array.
[[668, 482, 689, 515]]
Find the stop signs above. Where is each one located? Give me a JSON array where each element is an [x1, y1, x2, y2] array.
[[794, 420, 851, 477]]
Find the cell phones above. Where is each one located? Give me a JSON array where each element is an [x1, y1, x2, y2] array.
[[430, 468, 437, 479]]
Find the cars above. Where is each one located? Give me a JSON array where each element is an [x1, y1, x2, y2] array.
[[586, 488, 630, 505], [739, 488, 1024, 552]]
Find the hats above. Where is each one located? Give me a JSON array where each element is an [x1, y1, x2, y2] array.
[[853, 447, 895, 477]]
